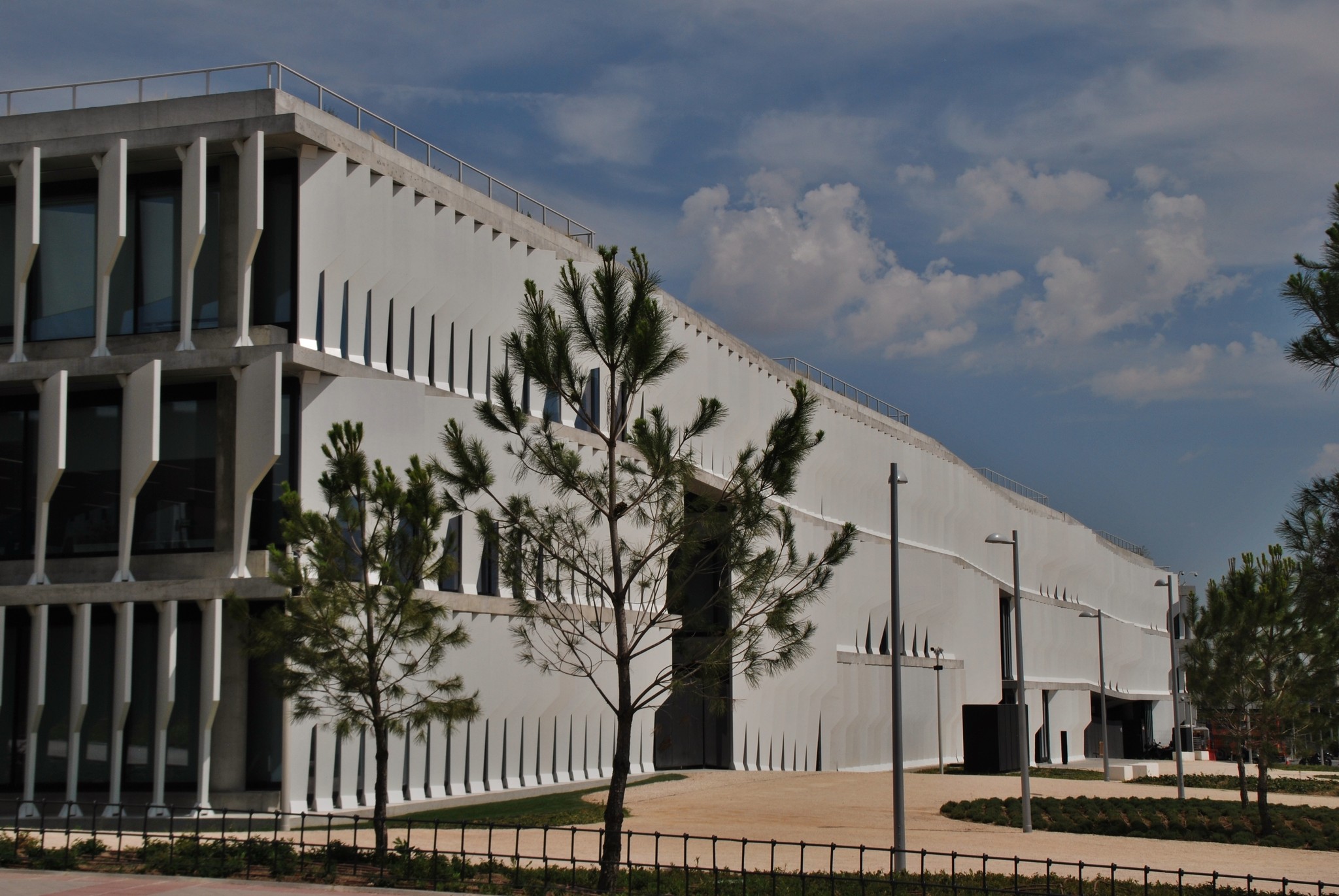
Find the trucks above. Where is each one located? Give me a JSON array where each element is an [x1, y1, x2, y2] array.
[[1193, 712, 1287, 762]]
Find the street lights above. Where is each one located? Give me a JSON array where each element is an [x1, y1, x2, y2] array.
[[1077, 609, 1111, 782], [1153, 573, 1185, 799], [887, 462, 909, 871], [984, 530, 1033, 833]]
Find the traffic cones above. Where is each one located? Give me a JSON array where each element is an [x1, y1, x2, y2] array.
[[1285, 756, 1290, 765]]
